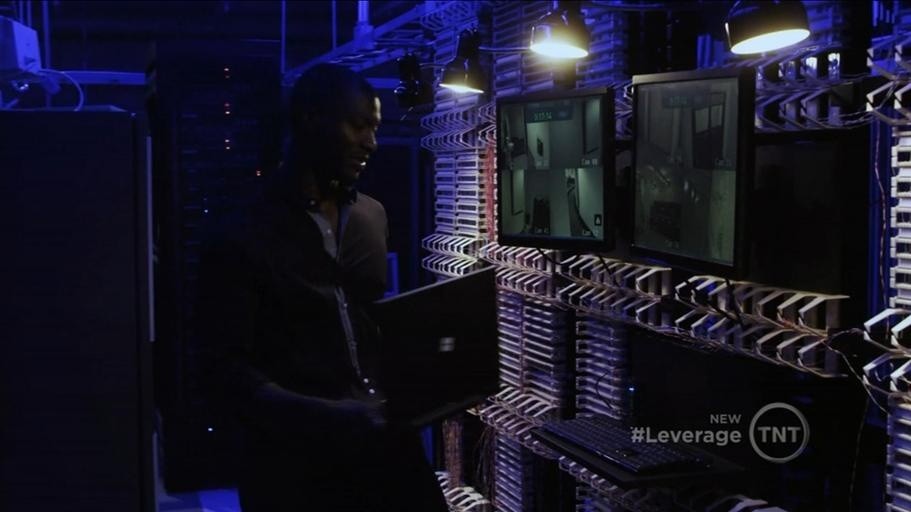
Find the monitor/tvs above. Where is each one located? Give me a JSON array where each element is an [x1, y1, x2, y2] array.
[[496, 85, 618, 253], [629, 68, 755, 277]]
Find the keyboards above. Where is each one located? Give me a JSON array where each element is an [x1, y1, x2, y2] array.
[[542, 415, 695, 474]]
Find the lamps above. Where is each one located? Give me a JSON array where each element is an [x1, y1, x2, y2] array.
[[392, 0, 812, 109]]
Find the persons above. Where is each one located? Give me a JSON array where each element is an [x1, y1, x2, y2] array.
[[227, 63, 457, 512]]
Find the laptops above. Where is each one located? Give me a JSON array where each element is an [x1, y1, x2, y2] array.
[[366, 258, 502, 430]]
